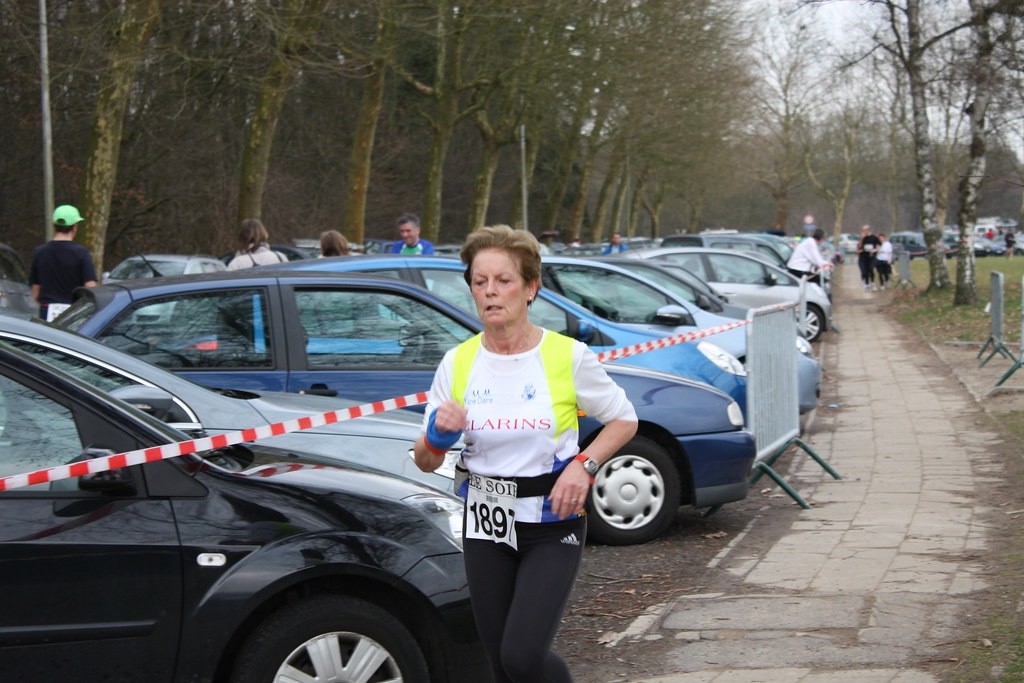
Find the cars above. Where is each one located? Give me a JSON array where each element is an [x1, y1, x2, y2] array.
[[218, 236, 465, 270], [828, 233, 861, 253], [0, 340, 496, 683], [48, 272, 757, 545], [942, 232, 1005, 258], [233, 257, 745, 427], [1, 315, 468, 505], [536, 247, 831, 413], [548, 236, 664, 256]]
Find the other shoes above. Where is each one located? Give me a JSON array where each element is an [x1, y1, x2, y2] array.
[[865, 284, 869, 289], [880, 286, 884, 290], [871, 283, 877, 291]]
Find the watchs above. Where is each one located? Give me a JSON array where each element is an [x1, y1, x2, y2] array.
[[573, 453, 598, 484]]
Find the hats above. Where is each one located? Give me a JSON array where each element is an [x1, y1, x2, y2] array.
[[53, 205, 85, 226]]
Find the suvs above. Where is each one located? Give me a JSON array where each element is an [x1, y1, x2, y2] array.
[[888, 230, 952, 260], [0, 242, 44, 321], [101, 252, 228, 286]]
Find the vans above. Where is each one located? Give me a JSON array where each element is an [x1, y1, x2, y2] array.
[[661, 233, 794, 267]]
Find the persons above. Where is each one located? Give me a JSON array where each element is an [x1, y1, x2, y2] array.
[[599, 232, 628, 256], [861, 225, 882, 291], [414, 223, 639, 683], [857, 234, 865, 270], [998, 226, 1004, 235], [225, 218, 290, 273], [391, 213, 433, 258], [1004, 230, 1016, 262], [317, 229, 364, 257], [875, 233, 893, 291], [565, 238, 582, 248], [787, 228, 835, 303], [30, 205, 99, 322], [985, 228, 994, 240]]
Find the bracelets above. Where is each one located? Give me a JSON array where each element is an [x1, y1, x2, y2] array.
[[424, 436, 448, 456]]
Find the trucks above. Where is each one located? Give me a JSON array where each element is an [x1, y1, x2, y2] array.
[[964, 216, 1024, 256]]
